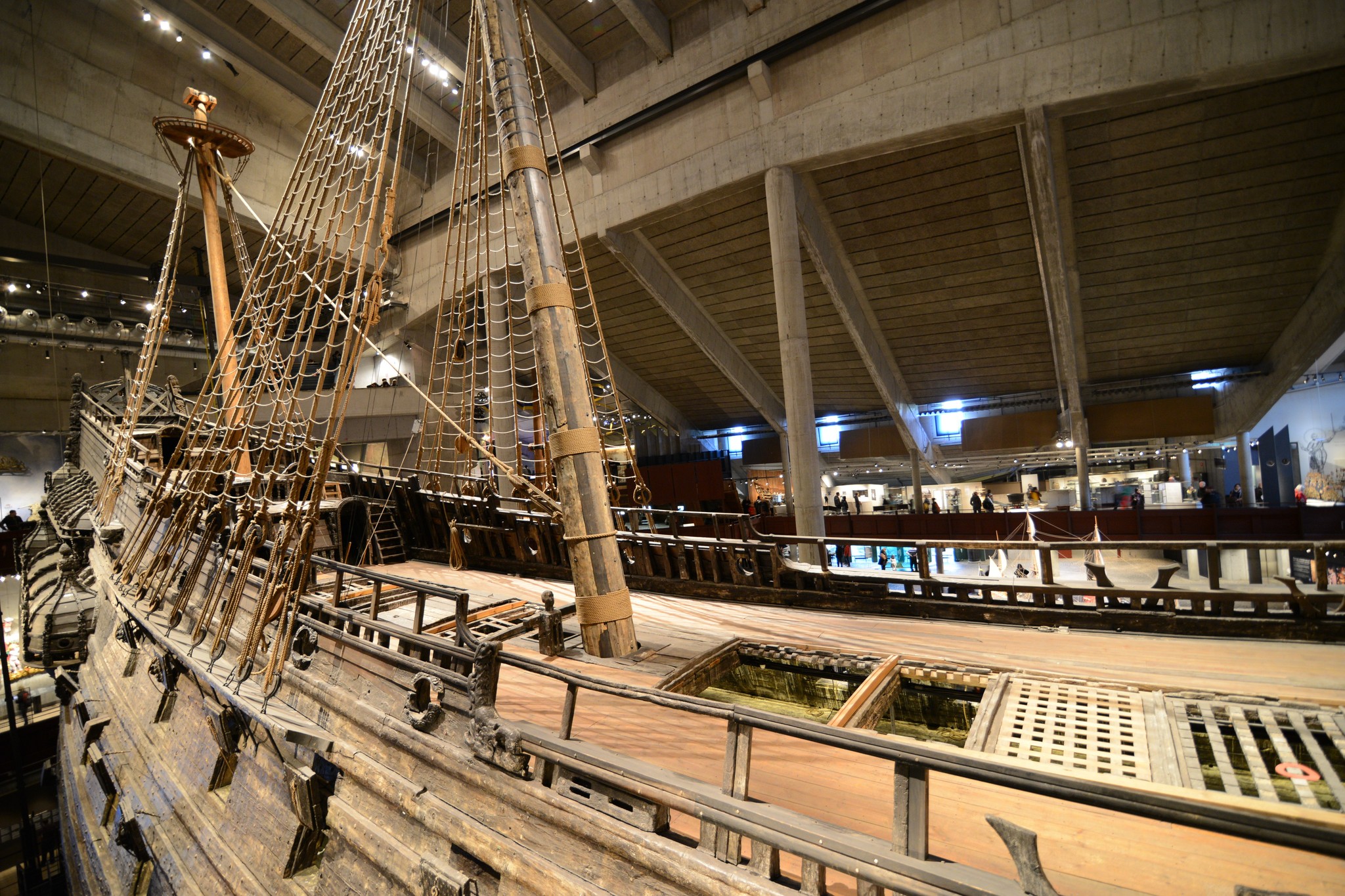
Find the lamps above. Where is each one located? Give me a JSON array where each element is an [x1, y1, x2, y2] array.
[[404, 340, 412, 350], [161, 304, 166, 310], [1304, 373, 1342, 384], [1224, 449, 1230, 453], [118, 294, 126, 305], [1036, 457, 1058, 467], [750, 479, 753, 482], [755, 477, 758, 481], [1056, 433, 1063, 447], [36, 283, 47, 295], [179, 305, 187, 314], [26, 279, 32, 289], [328, 304, 333, 311], [1063, 433, 1072, 448]]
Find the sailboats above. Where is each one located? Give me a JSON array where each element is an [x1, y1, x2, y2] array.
[[782, 498, 1179, 610], [16, 0, 1345, 896]]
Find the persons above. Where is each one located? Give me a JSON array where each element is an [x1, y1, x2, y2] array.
[[738, 483, 995, 573], [1196, 481, 1208, 499], [1199, 485, 1224, 510], [1131, 486, 1144, 511], [1254, 482, 1263, 506], [332, 351, 341, 364], [313, 368, 321, 376], [0, 509, 22, 533], [366, 377, 398, 390], [1014, 565, 1029, 578], [15, 685, 31, 727], [330, 383, 337, 390], [307, 359, 316, 365], [271, 369, 292, 378], [1229, 484, 1243, 505], [1293, 484, 1307, 506], [1168, 476, 1176, 483], [1325, 565, 1338, 587], [347, 379, 356, 389]]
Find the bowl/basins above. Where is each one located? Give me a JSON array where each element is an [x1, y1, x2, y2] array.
[[1007, 493, 1025, 503]]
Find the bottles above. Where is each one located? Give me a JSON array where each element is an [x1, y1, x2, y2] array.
[[955, 495, 957, 499], [896, 493, 898, 496]]
